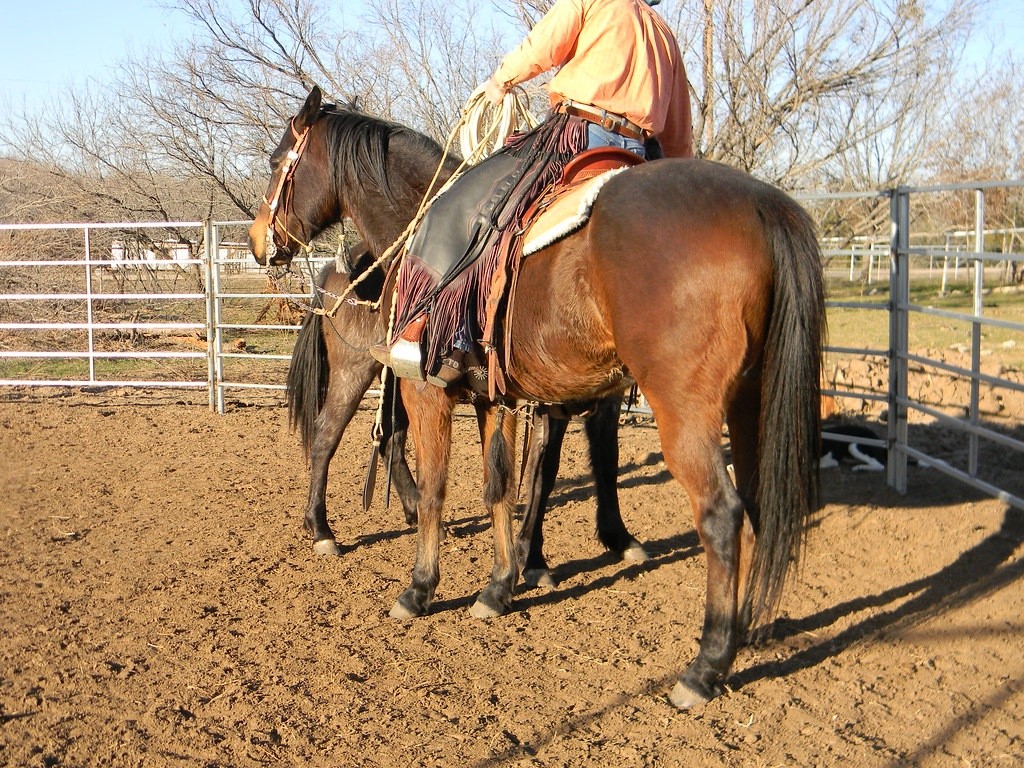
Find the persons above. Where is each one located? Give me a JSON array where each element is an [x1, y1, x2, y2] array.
[[369, 0, 695, 387]]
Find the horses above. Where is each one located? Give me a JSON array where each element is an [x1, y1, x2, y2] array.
[[244, 78, 829, 714]]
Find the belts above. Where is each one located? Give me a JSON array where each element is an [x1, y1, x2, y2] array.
[[554, 106, 645, 146]]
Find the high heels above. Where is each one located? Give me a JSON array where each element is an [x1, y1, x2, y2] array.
[[369, 345, 466, 388]]
